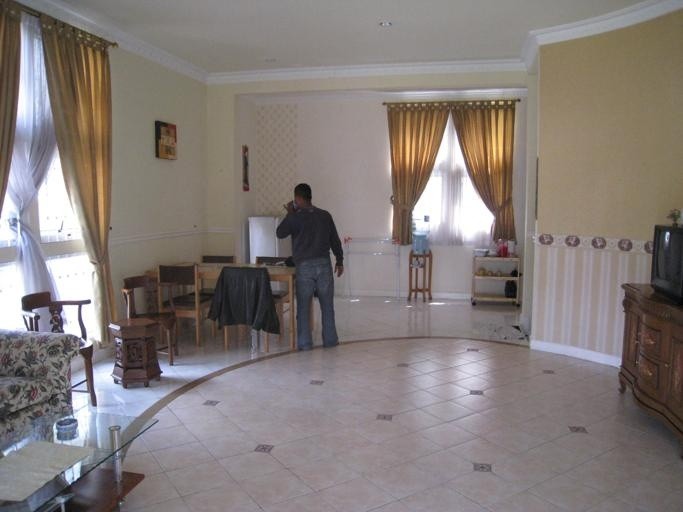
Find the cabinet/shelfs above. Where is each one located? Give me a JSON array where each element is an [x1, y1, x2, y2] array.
[[616, 281, 682, 447], [470, 253, 521, 305]]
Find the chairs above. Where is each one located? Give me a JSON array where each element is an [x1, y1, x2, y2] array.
[[0, 326, 84, 451], [107, 256, 315, 389], [20, 289, 100, 407]]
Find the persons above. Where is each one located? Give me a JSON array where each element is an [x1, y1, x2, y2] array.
[[276, 183, 344, 352]]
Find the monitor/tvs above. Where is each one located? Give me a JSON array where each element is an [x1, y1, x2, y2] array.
[[649, 224, 683, 308]]
